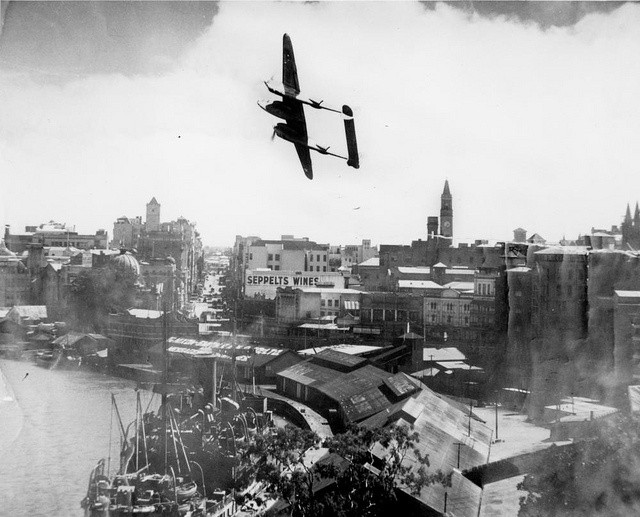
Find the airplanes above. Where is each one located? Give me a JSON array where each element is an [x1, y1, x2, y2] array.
[[257, 32, 360, 179]]
